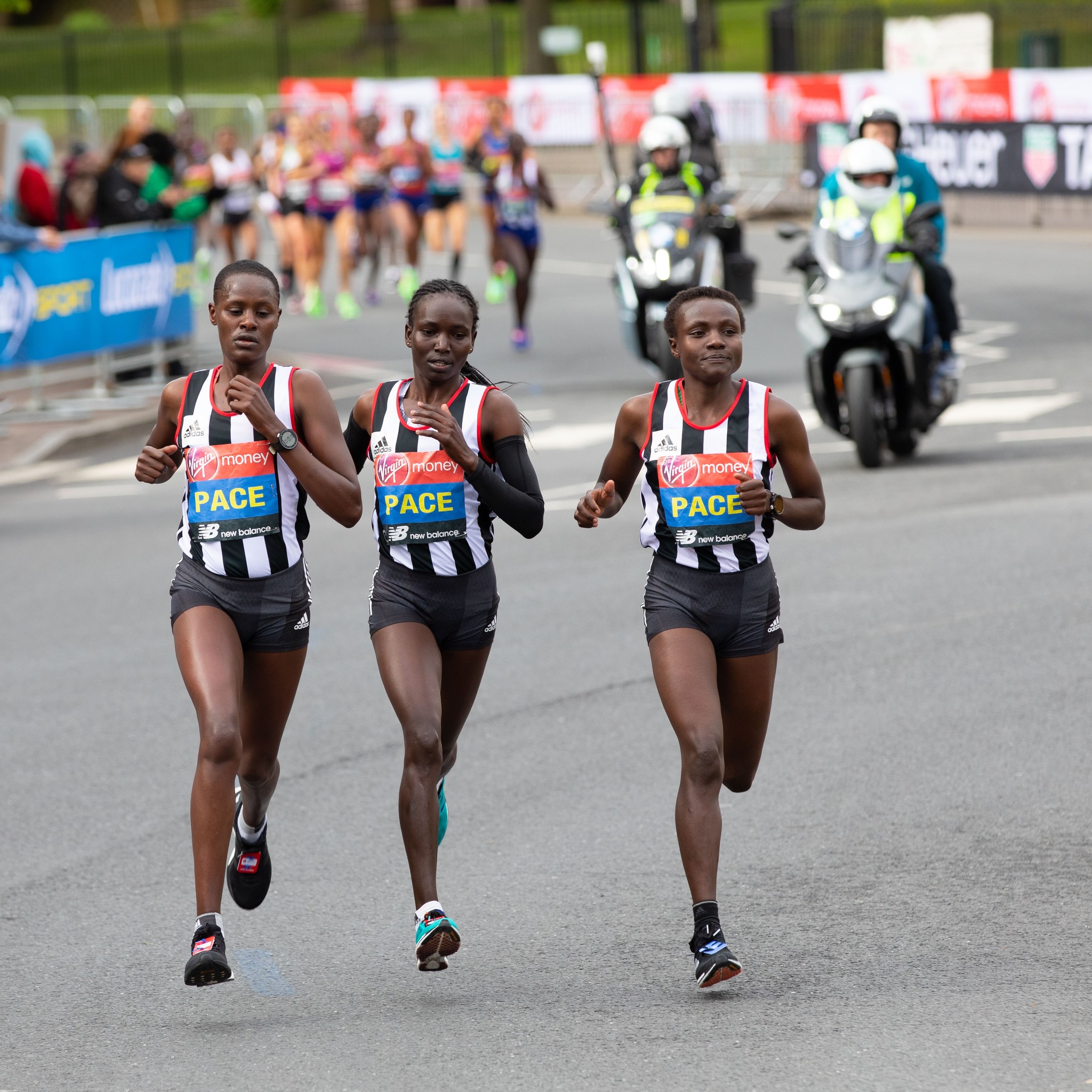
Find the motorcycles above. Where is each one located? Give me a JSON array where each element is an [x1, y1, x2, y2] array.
[[775, 201, 964, 470], [604, 182, 755, 379]]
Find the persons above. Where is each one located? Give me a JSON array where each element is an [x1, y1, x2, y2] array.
[[135, 259, 363, 986], [343, 280, 546, 971], [1, 100, 958, 379], [574, 287, 826, 987]]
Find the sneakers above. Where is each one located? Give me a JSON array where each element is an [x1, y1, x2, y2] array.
[[415, 909, 461, 972], [227, 786, 272, 911], [437, 777, 449, 847], [184, 922, 235, 988], [688, 921, 742, 989]]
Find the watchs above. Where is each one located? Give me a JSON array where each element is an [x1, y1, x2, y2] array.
[[268, 428, 299, 454], [763, 489, 784, 520]]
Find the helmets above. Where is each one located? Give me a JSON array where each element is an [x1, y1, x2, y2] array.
[[849, 100, 910, 147], [638, 115, 689, 165], [836, 138, 901, 210]]
